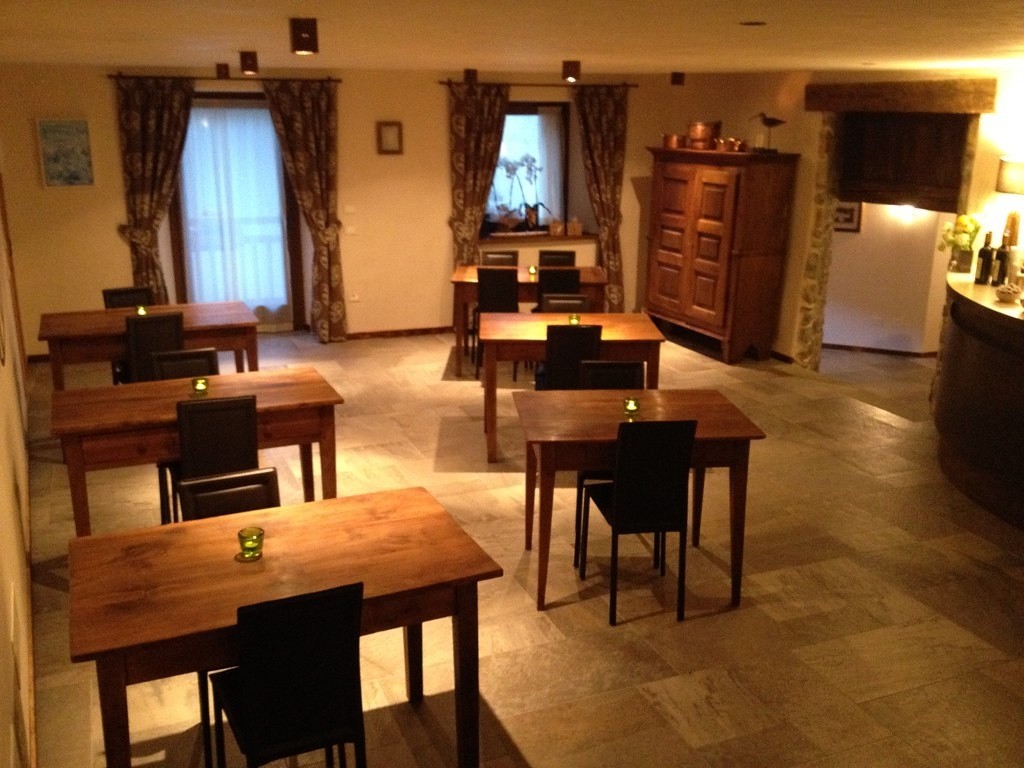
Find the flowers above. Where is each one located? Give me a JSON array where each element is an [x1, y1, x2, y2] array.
[[491, 156, 555, 218]]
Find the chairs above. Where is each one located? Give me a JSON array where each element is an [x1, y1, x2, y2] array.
[[101, 287, 367, 768], [469, 250, 697, 626]]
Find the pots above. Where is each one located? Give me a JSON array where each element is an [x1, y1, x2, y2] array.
[[660, 133, 688, 148], [687, 119, 722, 150]]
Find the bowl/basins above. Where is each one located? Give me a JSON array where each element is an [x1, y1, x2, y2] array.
[[995, 291, 1022, 303]]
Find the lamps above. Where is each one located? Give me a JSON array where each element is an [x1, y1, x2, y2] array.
[[239, 51, 259, 74], [562, 60, 580, 82], [995, 159, 1024, 195], [289, 17, 319, 55]]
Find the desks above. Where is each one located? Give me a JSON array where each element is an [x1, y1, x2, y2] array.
[[513, 389, 766, 612], [450, 266, 608, 377], [68, 487, 502, 768], [51, 367, 344, 536], [927, 271, 1024, 530], [479, 313, 666, 462], [38, 302, 260, 390]]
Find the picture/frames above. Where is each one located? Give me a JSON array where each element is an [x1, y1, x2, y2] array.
[[35, 118, 96, 189], [376, 120, 403, 155], [834, 202, 861, 232]]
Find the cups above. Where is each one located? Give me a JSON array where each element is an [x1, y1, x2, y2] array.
[[238, 526, 265, 560], [623, 397, 640, 415], [569, 314, 580, 325], [528, 264, 537, 275], [191, 378, 208, 394], [135, 305, 147, 316]]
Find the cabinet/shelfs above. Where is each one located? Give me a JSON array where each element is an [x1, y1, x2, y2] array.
[[644, 146, 800, 366]]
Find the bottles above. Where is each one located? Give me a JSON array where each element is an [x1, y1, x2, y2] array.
[[974, 233, 993, 286], [992, 233, 1010, 287]]
